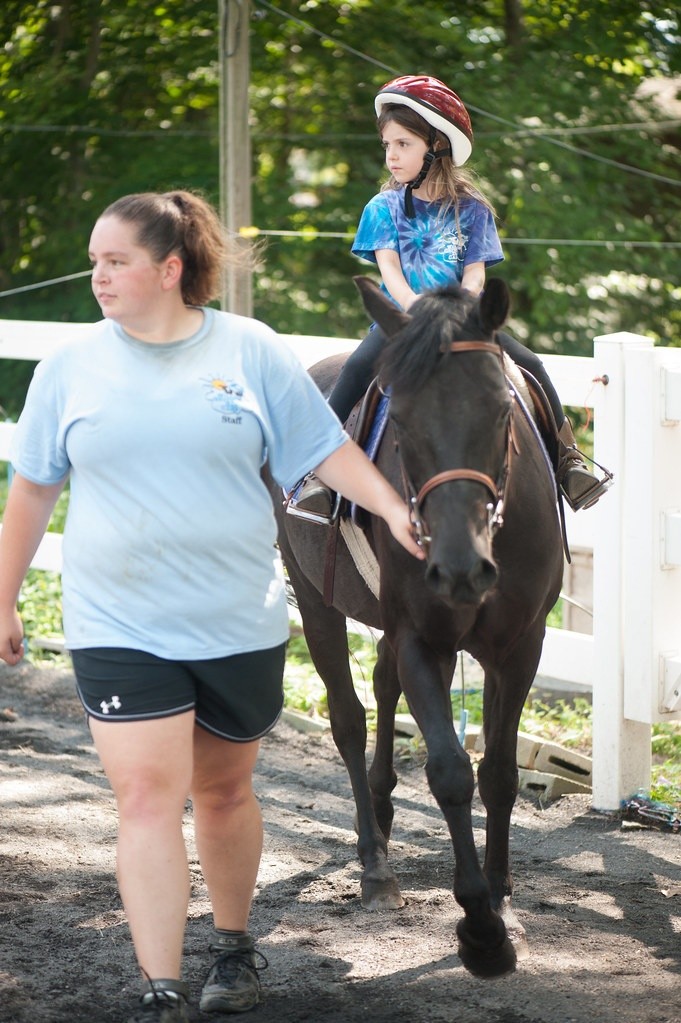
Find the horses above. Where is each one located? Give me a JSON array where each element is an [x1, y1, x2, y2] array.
[[261, 271, 566, 979]]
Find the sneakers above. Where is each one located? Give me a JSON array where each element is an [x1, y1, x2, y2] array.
[[128, 965, 193, 1023], [198, 931, 269, 1015]]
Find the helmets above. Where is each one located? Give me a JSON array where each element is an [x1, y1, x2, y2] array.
[[375, 74, 473, 168]]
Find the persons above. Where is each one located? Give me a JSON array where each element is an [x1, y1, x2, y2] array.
[[0, 186, 429, 1023], [291, 75, 607, 519]]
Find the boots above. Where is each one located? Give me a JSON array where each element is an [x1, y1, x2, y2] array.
[[553, 414, 601, 510], [293, 472, 333, 517]]
